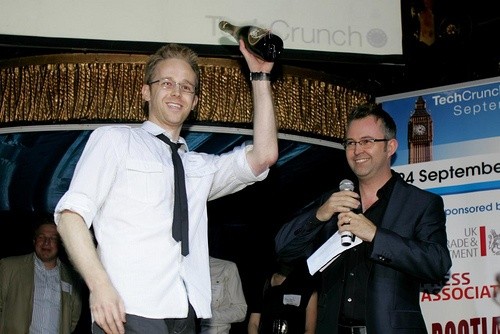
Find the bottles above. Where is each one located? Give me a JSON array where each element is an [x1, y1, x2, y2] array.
[[218, 21, 283, 62]]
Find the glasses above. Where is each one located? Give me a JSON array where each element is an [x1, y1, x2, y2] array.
[[36, 233, 60, 243], [150, 77, 199, 93], [343, 138, 389, 150]]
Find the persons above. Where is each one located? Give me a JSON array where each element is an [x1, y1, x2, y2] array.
[[274, 104, 451, 334], [202, 257, 248, 334], [248, 262, 317, 334], [53, 40, 279, 334], [0, 223, 82, 334]]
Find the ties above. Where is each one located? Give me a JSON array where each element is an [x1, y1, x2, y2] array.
[[156, 133, 189, 256]]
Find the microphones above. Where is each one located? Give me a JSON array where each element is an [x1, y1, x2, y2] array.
[[339, 179, 354, 246]]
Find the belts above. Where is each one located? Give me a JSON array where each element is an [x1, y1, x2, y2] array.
[[338, 326, 367, 334]]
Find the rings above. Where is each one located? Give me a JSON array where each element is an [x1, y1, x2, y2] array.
[[348, 216, 352, 223]]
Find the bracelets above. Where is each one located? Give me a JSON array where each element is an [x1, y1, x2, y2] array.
[[250, 71, 272, 82]]
